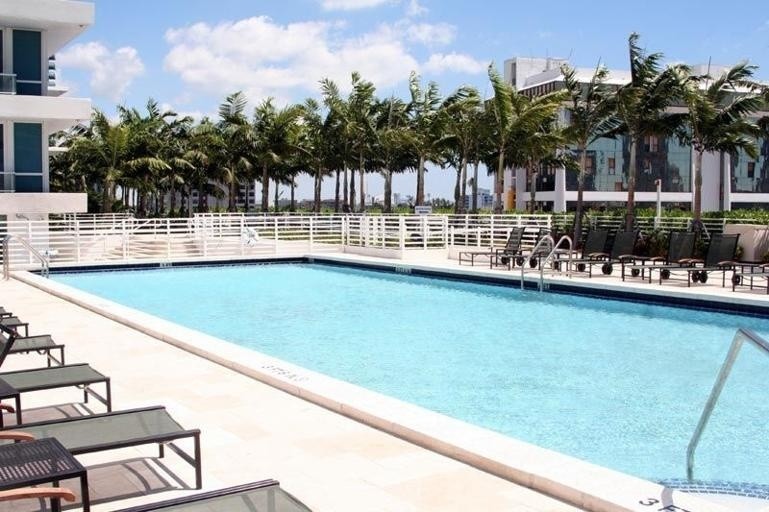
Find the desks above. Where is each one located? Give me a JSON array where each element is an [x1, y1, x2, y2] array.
[[1, 380, 22, 429], [1, 436, 89, 511]]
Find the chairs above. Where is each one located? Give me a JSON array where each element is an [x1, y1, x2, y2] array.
[[1, 334, 65, 369], [2, 363, 111, 414], [458, 223, 741, 288], [1, 406, 201, 510], [0, 296, 29, 354], [114, 479, 311, 510]]
